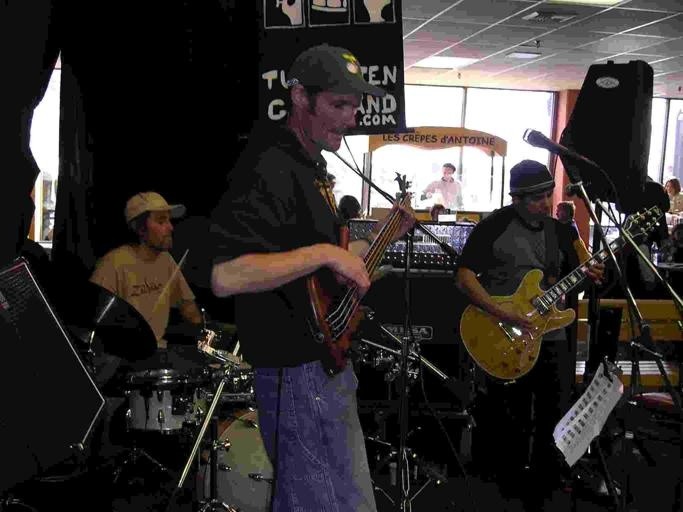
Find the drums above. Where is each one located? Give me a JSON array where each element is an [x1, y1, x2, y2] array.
[[124, 370, 192, 431], [209, 363, 255, 403], [194, 409, 273, 511]]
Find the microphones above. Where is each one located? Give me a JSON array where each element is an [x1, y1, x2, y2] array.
[[629, 341, 663, 362], [564, 182, 581, 197], [523, 128, 598, 171]]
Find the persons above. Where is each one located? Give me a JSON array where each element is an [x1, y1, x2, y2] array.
[[336, 195, 362, 221], [448, 159, 604, 497], [420, 162, 465, 213], [669, 225, 682, 264], [555, 200, 580, 244], [662, 178, 682, 214], [86, 189, 202, 353], [429, 203, 443, 219], [205, 44, 416, 511]]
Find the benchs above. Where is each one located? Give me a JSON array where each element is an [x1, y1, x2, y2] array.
[[573, 297, 682, 388]]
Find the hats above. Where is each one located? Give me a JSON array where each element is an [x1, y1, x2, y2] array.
[[287, 43, 387, 99], [509, 160, 555, 195], [124, 192, 186, 222]]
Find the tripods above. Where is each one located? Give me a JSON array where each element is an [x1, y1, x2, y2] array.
[[371, 237, 448, 512], [196, 431, 237, 512]]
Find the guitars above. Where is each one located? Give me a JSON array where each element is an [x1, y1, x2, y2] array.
[[460, 205, 663, 381], [307, 172, 410, 373]]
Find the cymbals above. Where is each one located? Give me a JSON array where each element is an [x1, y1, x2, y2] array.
[[50, 281, 157, 360]]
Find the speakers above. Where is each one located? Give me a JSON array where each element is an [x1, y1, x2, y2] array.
[[557, 59, 652, 210]]
